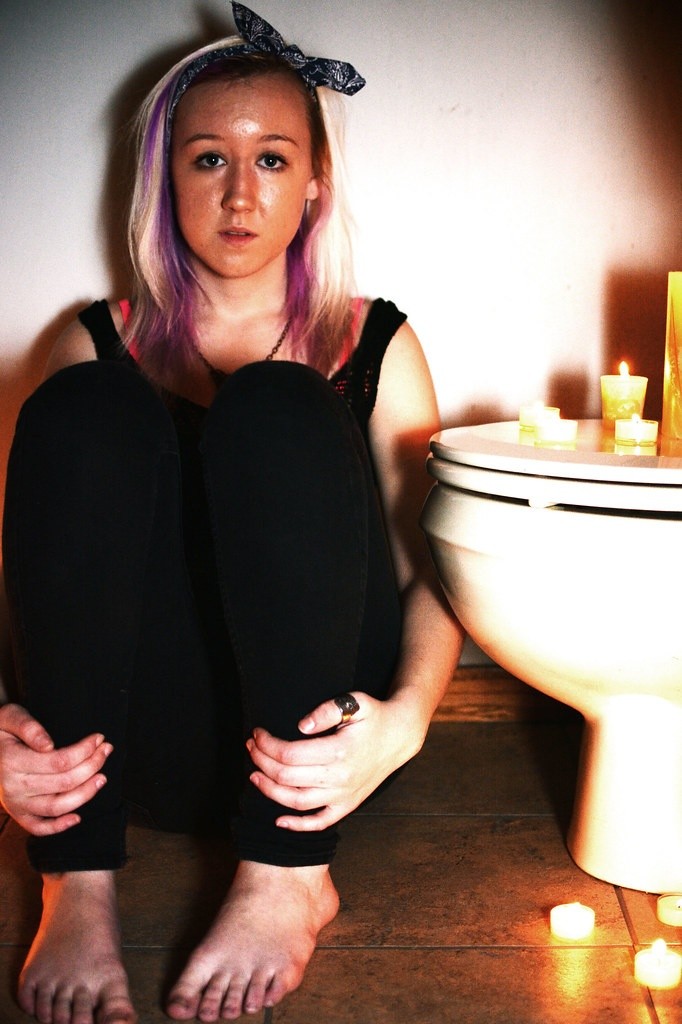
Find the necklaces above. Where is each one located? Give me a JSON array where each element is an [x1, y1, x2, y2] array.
[[192, 322, 290, 385]]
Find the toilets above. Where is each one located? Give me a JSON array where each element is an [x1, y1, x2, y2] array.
[[425, 411, 682, 891]]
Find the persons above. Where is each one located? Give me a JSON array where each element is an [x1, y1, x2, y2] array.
[[0, 0, 466, 1024]]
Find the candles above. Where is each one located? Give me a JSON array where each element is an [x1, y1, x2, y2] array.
[[549, 902, 595, 941], [657, 892, 682, 927], [519, 397, 579, 451], [615, 414, 659, 457], [601, 361, 649, 430], [634, 938, 682, 989]]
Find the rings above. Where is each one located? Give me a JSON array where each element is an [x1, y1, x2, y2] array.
[[334, 693, 360, 723]]
